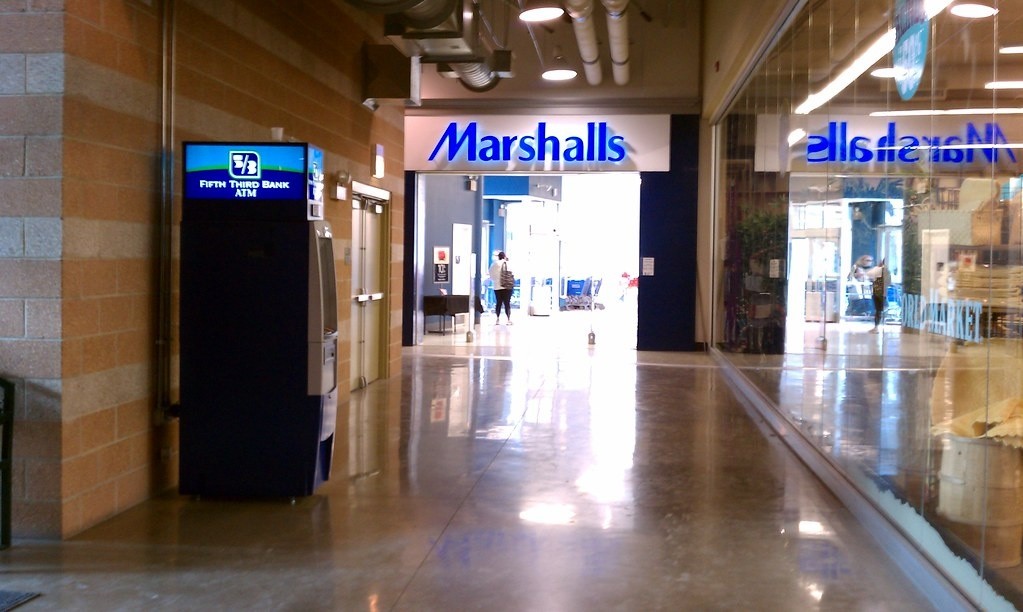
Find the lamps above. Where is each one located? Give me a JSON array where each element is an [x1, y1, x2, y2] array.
[[541, 43, 577, 82], [516, 0, 564, 23], [329, 170, 352, 200], [467, 176, 479, 192]]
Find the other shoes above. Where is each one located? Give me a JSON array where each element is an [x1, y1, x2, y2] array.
[[869, 328, 879, 334], [506, 320, 514, 325], [496, 320, 499, 325]]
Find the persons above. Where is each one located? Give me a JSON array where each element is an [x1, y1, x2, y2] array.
[[489, 252, 514, 325], [850, 255, 873, 316], [866, 259, 884, 333]]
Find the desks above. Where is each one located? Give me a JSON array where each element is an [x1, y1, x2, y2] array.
[[423, 295, 484, 336]]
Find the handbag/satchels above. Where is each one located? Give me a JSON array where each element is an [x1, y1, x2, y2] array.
[[500, 263, 513, 288]]
